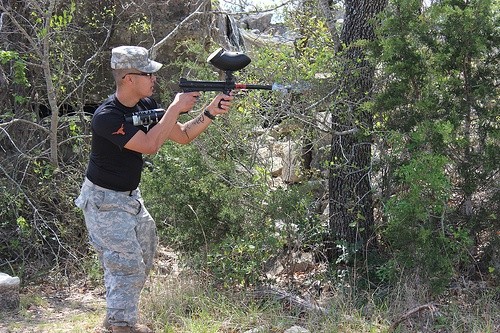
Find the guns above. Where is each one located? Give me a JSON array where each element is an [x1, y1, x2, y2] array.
[[124, 48, 272, 132]]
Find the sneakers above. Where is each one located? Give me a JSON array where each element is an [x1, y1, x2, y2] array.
[[103, 316, 152, 333], [111, 326, 132, 333]]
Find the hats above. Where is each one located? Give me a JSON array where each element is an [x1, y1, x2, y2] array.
[[111, 46, 163, 73]]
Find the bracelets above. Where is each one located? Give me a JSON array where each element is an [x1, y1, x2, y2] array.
[[204, 108, 216, 120]]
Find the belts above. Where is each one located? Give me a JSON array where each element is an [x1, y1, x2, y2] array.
[[84, 177, 139, 196]]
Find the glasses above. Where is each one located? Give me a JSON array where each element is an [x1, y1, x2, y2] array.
[[121, 72, 152, 79]]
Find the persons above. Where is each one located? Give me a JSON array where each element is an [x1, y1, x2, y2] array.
[[73, 44, 235, 333]]
